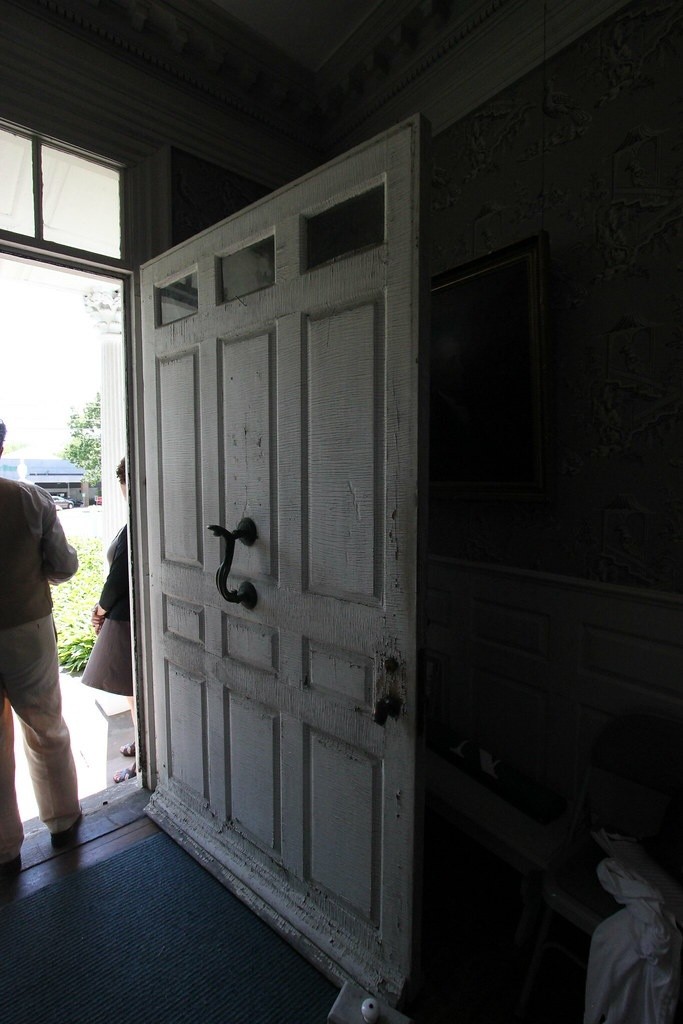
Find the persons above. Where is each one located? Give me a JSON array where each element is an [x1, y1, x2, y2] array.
[[0, 418, 82, 878], [81, 456, 137, 784]]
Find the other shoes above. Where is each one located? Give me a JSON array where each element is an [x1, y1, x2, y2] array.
[[113, 768, 136, 783], [119, 739, 135, 756], [50, 805, 83, 848], [0, 853, 22, 876]]
